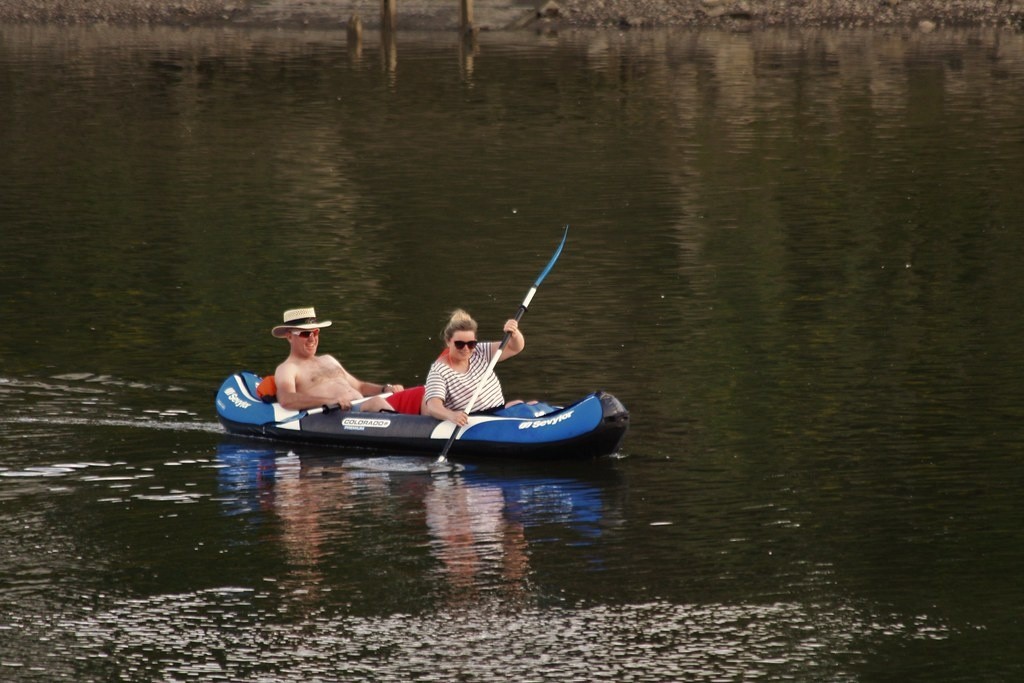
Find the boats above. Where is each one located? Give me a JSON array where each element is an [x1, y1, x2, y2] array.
[[215, 372, 630, 459]]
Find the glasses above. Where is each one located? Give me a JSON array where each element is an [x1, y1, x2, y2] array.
[[290, 329, 320, 338], [454, 339, 477, 350]]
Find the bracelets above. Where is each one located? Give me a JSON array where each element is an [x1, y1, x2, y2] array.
[[381, 384, 392, 393]]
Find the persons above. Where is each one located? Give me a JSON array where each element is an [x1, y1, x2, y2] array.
[[271, 307, 404, 413], [423, 308, 539, 427]]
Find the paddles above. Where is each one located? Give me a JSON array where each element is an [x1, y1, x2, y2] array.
[[258, 392, 394, 428], [437, 223, 570, 462]]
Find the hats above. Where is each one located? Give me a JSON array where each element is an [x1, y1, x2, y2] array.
[[271, 307, 332, 338]]
